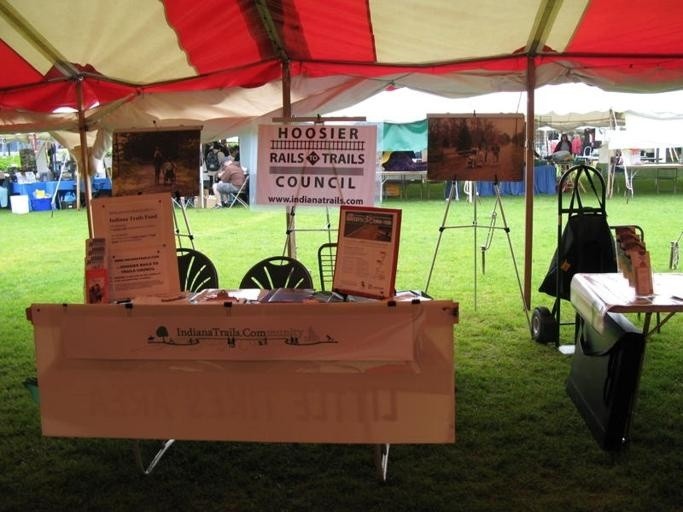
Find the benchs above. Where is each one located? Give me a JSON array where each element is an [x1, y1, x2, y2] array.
[[26, 301, 458, 485]]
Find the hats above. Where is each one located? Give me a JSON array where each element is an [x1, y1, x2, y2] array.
[[218, 157, 230, 166]]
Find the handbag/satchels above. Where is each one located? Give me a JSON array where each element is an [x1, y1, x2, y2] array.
[[566, 311, 644, 451], [539, 211, 618, 302]]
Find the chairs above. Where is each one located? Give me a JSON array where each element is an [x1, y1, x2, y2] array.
[[229, 176, 249, 209], [656, 168, 677, 195], [609, 225, 660, 334], [318, 243, 337, 291], [239, 256, 313, 289], [176, 248, 218, 292]]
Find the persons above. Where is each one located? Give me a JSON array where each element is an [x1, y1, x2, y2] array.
[[162, 159, 177, 186], [221, 138, 240, 161], [470, 144, 478, 168], [491, 142, 500, 162], [212, 156, 247, 208], [154, 145, 164, 184], [553, 134, 572, 155], [206, 144, 225, 194], [477, 143, 483, 167], [571, 135, 582, 158]]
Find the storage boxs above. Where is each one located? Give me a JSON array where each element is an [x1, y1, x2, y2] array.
[[30, 198, 52, 210]]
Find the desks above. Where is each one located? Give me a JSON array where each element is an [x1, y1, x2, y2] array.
[[476, 165, 556, 196], [110, 289, 433, 304], [13, 178, 112, 210], [616, 163, 682, 199], [569, 273, 682, 444], [379, 170, 430, 201], [552, 161, 587, 194]]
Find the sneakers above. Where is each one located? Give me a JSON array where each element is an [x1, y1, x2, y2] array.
[[213, 204, 222, 208], [222, 201, 229, 207]]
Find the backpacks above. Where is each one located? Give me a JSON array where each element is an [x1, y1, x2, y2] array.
[[205, 149, 221, 171]]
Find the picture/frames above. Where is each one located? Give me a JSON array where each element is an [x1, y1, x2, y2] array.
[[332, 205, 401, 300]]
